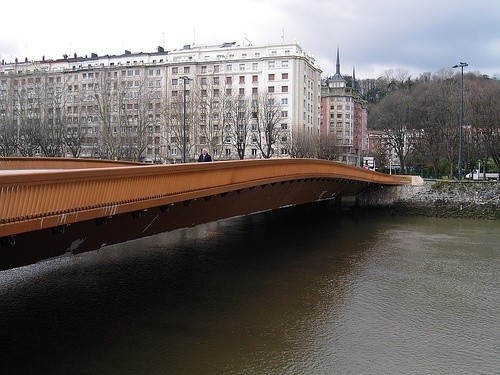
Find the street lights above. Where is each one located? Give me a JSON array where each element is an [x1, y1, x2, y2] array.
[[452, 62, 469, 179], [179, 76, 190, 163]]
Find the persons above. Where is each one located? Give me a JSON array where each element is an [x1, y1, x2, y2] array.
[[197, 148, 212, 162]]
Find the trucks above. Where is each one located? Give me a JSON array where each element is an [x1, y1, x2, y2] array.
[[465, 169, 499, 181]]
